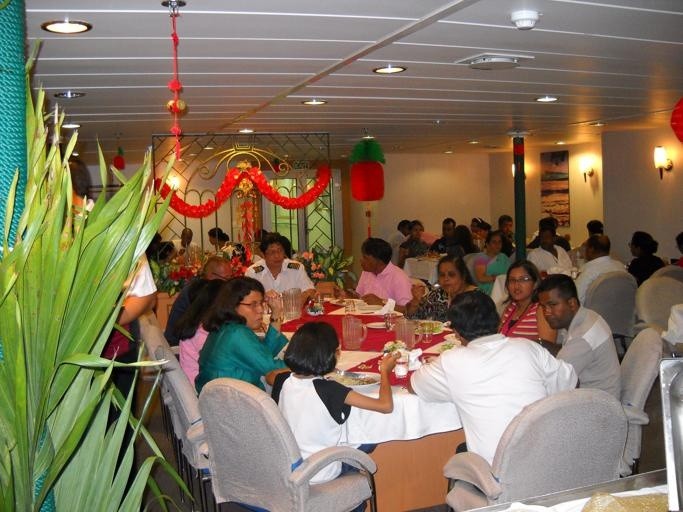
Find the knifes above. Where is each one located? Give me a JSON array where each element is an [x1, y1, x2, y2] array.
[[335, 369, 369, 379]]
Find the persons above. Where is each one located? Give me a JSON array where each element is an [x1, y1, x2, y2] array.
[[146, 213, 683, 512], [46, 157, 159, 512]]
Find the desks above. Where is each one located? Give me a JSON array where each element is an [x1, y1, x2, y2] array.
[[254, 287, 479, 510]]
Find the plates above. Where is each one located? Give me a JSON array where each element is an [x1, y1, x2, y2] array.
[[334, 370, 382, 390], [443, 333, 462, 344], [328, 298, 364, 306], [366, 321, 386, 329], [275, 330, 297, 361], [421, 341, 459, 354]]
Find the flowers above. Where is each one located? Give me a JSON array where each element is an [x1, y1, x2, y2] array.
[[148, 236, 357, 298]]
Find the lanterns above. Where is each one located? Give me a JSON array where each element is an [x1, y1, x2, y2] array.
[[274, 159, 280, 172], [348, 137, 386, 238], [670, 97, 683, 143], [113, 147, 125, 169], [167, 16, 185, 161]]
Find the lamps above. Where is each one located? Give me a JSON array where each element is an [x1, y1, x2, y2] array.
[[653, 146, 673, 179], [580, 160, 593, 183]]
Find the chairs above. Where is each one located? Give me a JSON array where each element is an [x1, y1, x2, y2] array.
[[604, 326, 665, 474], [138, 310, 210, 508], [489, 274, 509, 309], [651, 266, 683, 279], [203, 382, 378, 512], [447, 391, 629, 508], [636, 277, 682, 354], [583, 270, 637, 355]]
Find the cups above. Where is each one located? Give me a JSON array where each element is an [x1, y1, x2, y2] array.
[[316, 292, 325, 306], [345, 299, 356, 316], [281, 287, 302, 321], [341, 315, 368, 350], [384, 312, 398, 332], [394, 318, 423, 350], [570, 267, 578, 281], [393, 353, 410, 380], [420, 322, 434, 344]]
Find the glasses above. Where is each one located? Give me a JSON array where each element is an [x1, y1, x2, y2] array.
[[508, 275, 534, 284], [239, 299, 268, 310]]
